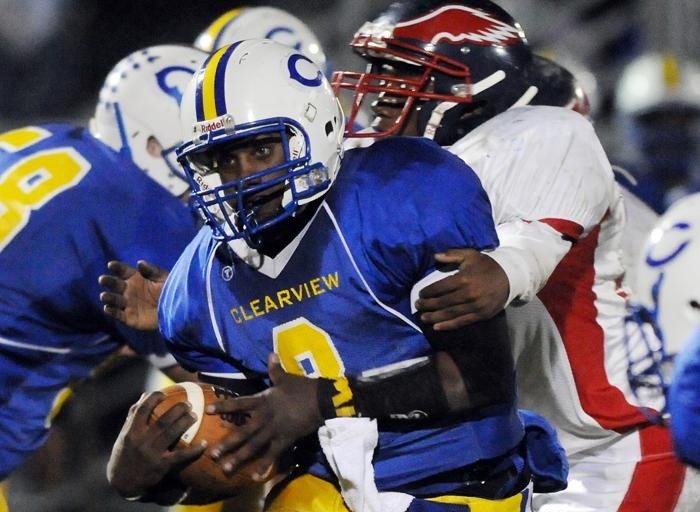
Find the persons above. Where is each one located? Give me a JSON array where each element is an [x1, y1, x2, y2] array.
[[0, 0, 700, 512]]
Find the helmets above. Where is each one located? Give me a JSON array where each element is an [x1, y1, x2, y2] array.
[[88, 42, 212, 196], [531, 53, 590, 118], [176, 37, 344, 242], [195, 8, 329, 80], [331, 1, 530, 148], [613, 51, 700, 116]]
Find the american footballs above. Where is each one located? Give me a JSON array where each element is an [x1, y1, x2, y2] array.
[[147, 380, 276, 491]]
